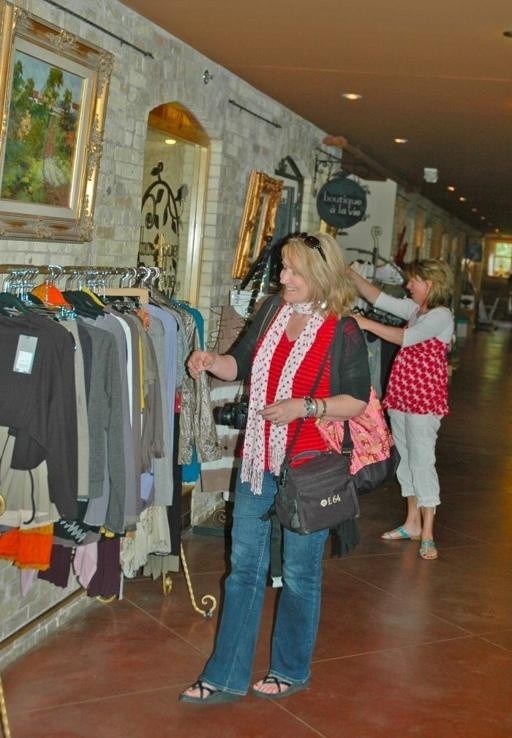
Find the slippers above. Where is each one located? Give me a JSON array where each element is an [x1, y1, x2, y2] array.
[[381, 527, 438, 559]]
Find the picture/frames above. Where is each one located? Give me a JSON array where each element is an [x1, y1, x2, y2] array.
[[231, 170, 284, 282], [0, 0, 115, 244]]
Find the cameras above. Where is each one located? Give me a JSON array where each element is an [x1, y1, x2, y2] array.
[[213, 398, 249, 429]]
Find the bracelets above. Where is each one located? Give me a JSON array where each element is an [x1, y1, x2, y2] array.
[[304, 395, 313, 419], [315, 399, 318, 417], [318, 398, 327, 419]]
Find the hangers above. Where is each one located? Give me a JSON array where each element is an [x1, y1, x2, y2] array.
[[1, 260, 166, 318]]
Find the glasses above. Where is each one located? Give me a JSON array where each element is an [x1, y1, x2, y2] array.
[[292, 231, 327, 263]]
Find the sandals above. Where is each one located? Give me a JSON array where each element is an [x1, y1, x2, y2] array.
[[252, 674, 307, 698], [179, 681, 240, 703]]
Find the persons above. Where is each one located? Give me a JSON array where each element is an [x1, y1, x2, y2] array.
[[343, 258, 456, 559], [179, 232, 371, 705]]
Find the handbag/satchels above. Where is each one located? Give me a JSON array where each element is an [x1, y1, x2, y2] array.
[[315, 386, 401, 492], [274, 451, 360, 535]]
